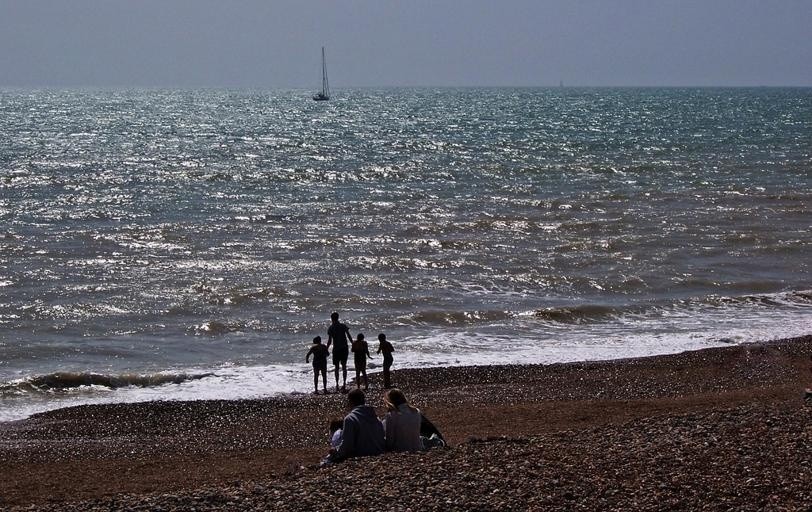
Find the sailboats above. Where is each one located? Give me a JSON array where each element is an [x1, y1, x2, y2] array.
[[312, 46, 330, 101]]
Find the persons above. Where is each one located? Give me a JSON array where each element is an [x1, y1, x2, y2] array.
[[306, 313, 421, 462]]
